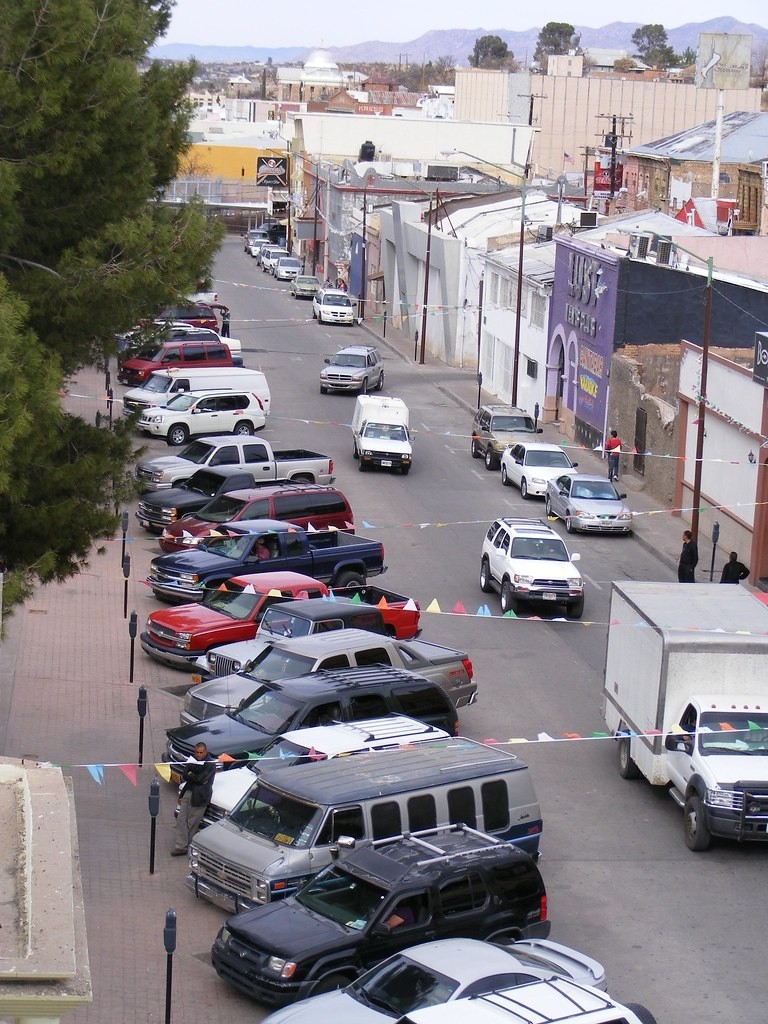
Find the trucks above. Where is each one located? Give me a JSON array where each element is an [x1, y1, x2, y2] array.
[[601, 580, 767, 853], [351, 394, 417, 476]]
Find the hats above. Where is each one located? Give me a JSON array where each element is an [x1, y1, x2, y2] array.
[[256, 538, 265, 544]]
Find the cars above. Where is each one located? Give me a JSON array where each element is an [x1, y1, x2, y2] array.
[[544, 472, 633, 537], [272, 256, 306, 281], [290, 274, 322, 300], [500, 441, 579, 500], [242, 222, 291, 273], [259, 937, 610, 1024]]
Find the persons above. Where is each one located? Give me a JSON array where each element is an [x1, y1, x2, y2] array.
[[254, 538, 270, 560], [337, 279, 348, 293], [170, 742, 216, 856], [220, 307, 230, 338], [678, 713, 702, 751], [385, 901, 414, 928], [605, 431, 622, 481], [678, 530, 698, 583], [719, 551, 750, 584], [235, 537, 245, 551]]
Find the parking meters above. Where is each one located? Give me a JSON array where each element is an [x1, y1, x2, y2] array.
[[710, 521, 720, 583], [358, 293, 361, 318], [121, 509, 129, 568], [383, 310, 387, 337], [137, 683, 148, 767], [128, 609, 139, 683], [104, 354, 111, 391], [122, 551, 132, 620], [477, 371, 483, 409], [108, 388, 113, 431], [415, 330, 419, 361], [163, 908, 177, 1024], [148, 778, 160, 874]]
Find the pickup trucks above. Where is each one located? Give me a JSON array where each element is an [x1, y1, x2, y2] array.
[[179, 623, 479, 725], [147, 518, 388, 605], [139, 570, 425, 677], [135, 434, 337, 496], [135, 464, 307, 535]]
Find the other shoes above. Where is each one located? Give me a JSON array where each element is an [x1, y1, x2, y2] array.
[[171, 847, 187, 856], [613, 475, 618, 481]]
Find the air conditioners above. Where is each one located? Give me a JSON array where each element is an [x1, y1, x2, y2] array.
[[571, 211, 598, 228], [628, 234, 649, 259], [657, 241, 675, 265]]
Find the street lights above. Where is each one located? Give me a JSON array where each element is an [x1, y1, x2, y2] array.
[[321, 161, 369, 320], [376, 171, 434, 364], [256, 145, 291, 256], [281, 150, 320, 278], [440, 147, 527, 407], [616, 225, 715, 544]]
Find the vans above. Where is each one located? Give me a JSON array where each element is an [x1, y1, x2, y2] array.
[[123, 367, 271, 417]]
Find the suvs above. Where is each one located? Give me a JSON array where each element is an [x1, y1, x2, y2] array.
[[210, 823, 552, 1011], [319, 344, 385, 397], [115, 290, 246, 387], [135, 385, 267, 447], [159, 482, 358, 554], [394, 974, 657, 1024], [160, 661, 460, 795], [471, 402, 543, 471], [479, 516, 587, 619], [191, 591, 398, 694], [174, 709, 453, 831], [312, 287, 357, 327], [184, 734, 544, 916]]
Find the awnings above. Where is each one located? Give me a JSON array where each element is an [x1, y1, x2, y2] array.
[[368, 271, 385, 282]]
